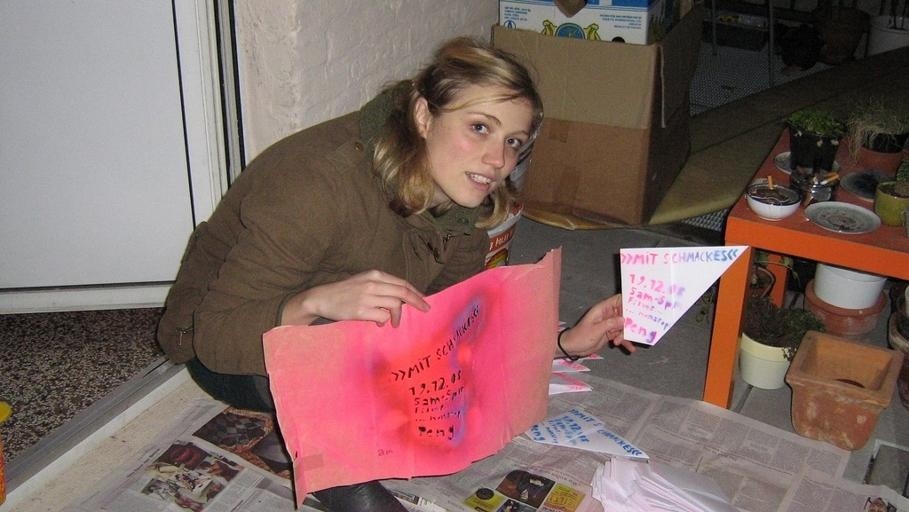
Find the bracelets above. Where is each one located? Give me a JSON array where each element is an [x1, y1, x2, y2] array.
[[556, 326, 580, 366]]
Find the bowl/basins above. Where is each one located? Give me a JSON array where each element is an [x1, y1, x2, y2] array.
[[743, 180, 801, 222]]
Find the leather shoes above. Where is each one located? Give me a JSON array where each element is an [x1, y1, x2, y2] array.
[[310, 478, 413, 512]]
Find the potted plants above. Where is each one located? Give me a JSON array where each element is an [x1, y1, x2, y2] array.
[[737, 296, 827, 392], [843, 95, 907, 180], [749, 247, 806, 303], [782, 106, 847, 170], [872, 153, 908, 230]]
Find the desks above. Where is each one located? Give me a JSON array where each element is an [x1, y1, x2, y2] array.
[[700, 102, 907, 412]]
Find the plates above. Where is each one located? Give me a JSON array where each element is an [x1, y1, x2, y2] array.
[[841, 167, 895, 201], [803, 198, 883, 235], [775, 148, 839, 180]]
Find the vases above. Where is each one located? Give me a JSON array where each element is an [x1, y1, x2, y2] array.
[[805, 277, 888, 339], [783, 325, 904, 450], [864, 15, 909, 60]]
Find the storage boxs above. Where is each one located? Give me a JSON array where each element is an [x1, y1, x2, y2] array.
[[487, 3, 707, 231], [496, 0, 655, 48]]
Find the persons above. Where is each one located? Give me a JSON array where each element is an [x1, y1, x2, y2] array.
[[155, 35, 636, 512]]
